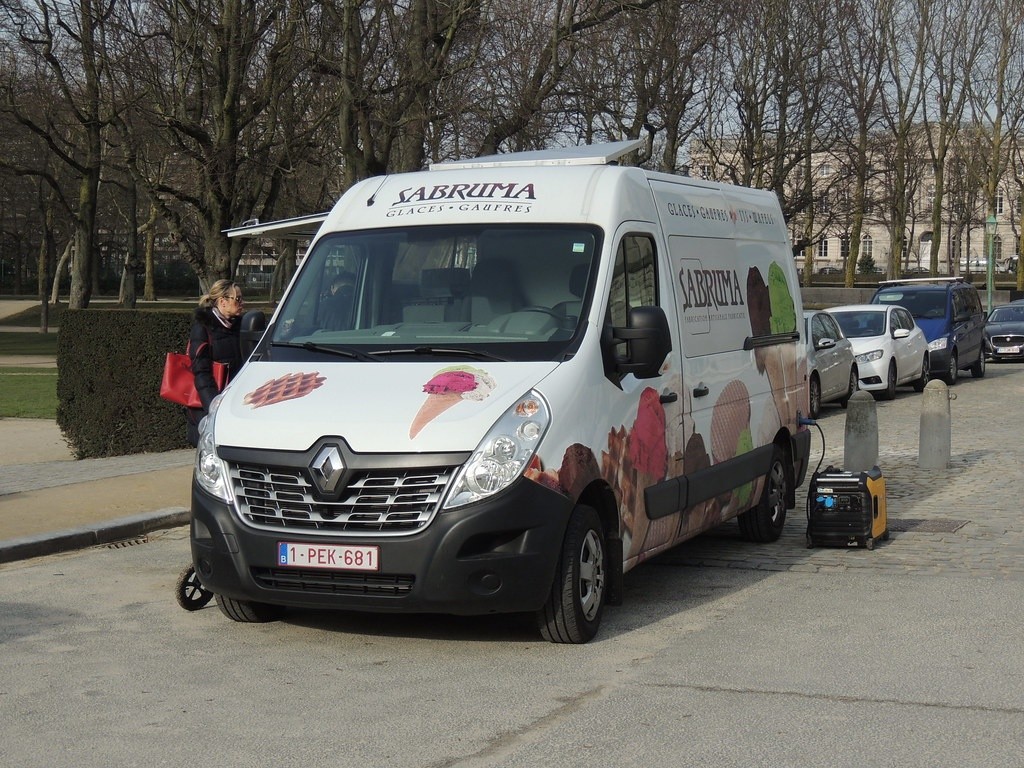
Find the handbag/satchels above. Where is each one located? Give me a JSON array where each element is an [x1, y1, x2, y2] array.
[[160, 323, 228, 408]]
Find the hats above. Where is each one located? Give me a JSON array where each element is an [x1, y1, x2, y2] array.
[[327, 272, 356, 297]]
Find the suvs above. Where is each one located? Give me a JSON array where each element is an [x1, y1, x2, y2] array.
[[860, 275, 986, 386]]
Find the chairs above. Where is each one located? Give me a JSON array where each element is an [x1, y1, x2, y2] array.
[[550, 264, 591, 327], [460, 258, 525, 322]]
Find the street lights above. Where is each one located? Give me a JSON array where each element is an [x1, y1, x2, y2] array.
[[984, 213, 998, 319]]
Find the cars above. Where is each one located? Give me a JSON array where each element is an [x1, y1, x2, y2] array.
[[983, 299, 1024, 363], [802, 309, 860, 421], [797, 259, 1006, 276], [822, 304, 930, 401]]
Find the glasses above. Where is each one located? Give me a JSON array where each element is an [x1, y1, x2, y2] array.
[[224, 296, 244, 303]]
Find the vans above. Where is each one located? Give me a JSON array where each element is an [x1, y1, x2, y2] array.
[[190, 140, 814, 645]]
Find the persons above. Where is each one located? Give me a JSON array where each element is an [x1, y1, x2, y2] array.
[[185, 279, 244, 449], [316, 271, 356, 331]]
